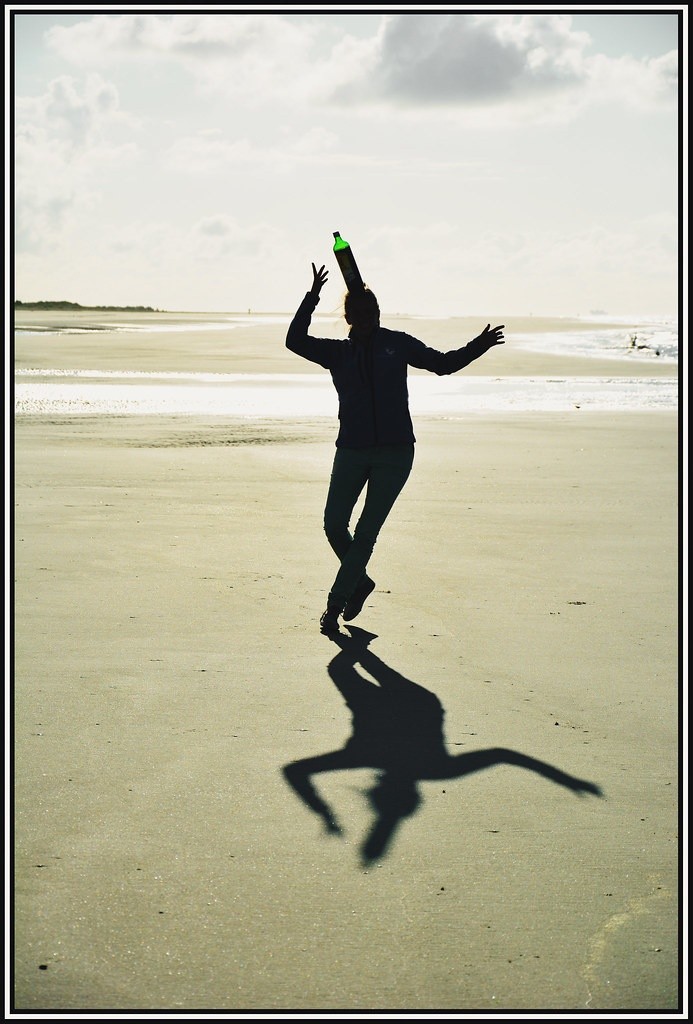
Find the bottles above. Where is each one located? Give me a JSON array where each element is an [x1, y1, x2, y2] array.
[[332, 230, 364, 291]]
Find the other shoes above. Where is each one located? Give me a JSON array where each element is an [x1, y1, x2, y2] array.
[[319, 593, 347, 631], [343, 569, 375, 621]]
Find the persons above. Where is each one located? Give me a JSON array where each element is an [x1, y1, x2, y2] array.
[[286, 262, 509, 635]]
